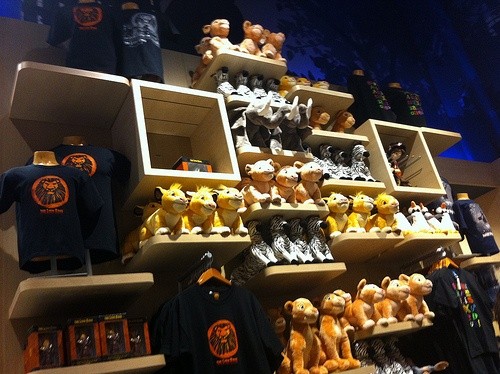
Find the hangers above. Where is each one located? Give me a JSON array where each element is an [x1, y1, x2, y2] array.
[[165, 253, 252, 305], [427, 247, 469, 279]]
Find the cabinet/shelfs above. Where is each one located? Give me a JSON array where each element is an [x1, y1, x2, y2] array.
[[8, 50, 500, 374]]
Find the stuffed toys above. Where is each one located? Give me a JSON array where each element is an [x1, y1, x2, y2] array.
[[120, 19, 461, 374]]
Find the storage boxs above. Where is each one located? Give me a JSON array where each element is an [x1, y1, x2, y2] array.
[[172, 156, 212, 173]]
